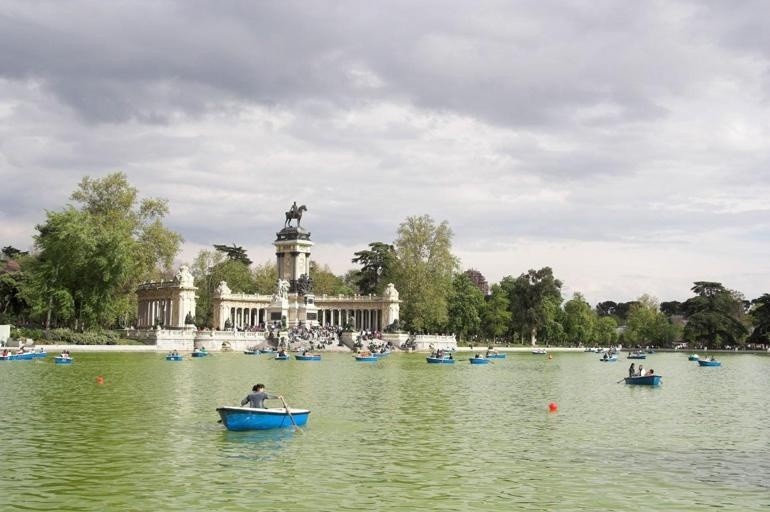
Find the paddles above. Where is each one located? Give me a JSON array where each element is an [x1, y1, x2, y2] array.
[[181, 353, 192, 361], [280, 396, 301, 433], [483, 357, 496, 366], [268, 355, 277, 360], [216, 403, 244, 425]]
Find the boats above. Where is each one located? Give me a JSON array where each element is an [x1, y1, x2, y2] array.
[[532, 348, 547, 355], [0, 349, 75, 366], [624, 374, 662, 388], [583, 346, 658, 363], [696, 358, 722, 367], [216, 404, 313, 433], [688, 351, 699, 361]]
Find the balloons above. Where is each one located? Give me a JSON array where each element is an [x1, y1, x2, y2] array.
[[549, 402, 558, 412]]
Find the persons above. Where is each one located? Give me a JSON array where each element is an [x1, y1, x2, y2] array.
[[645, 368, 655, 376], [239, 383, 283, 409], [577, 340, 722, 362], [723, 342, 770, 351], [431, 345, 500, 360], [1, 344, 70, 359], [168, 345, 207, 357], [289, 201, 298, 219], [629, 362, 636, 376], [535, 348, 547, 353], [635, 364, 647, 376], [236, 314, 396, 358]]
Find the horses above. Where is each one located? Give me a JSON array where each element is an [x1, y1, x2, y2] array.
[[285, 205, 307, 230]]
